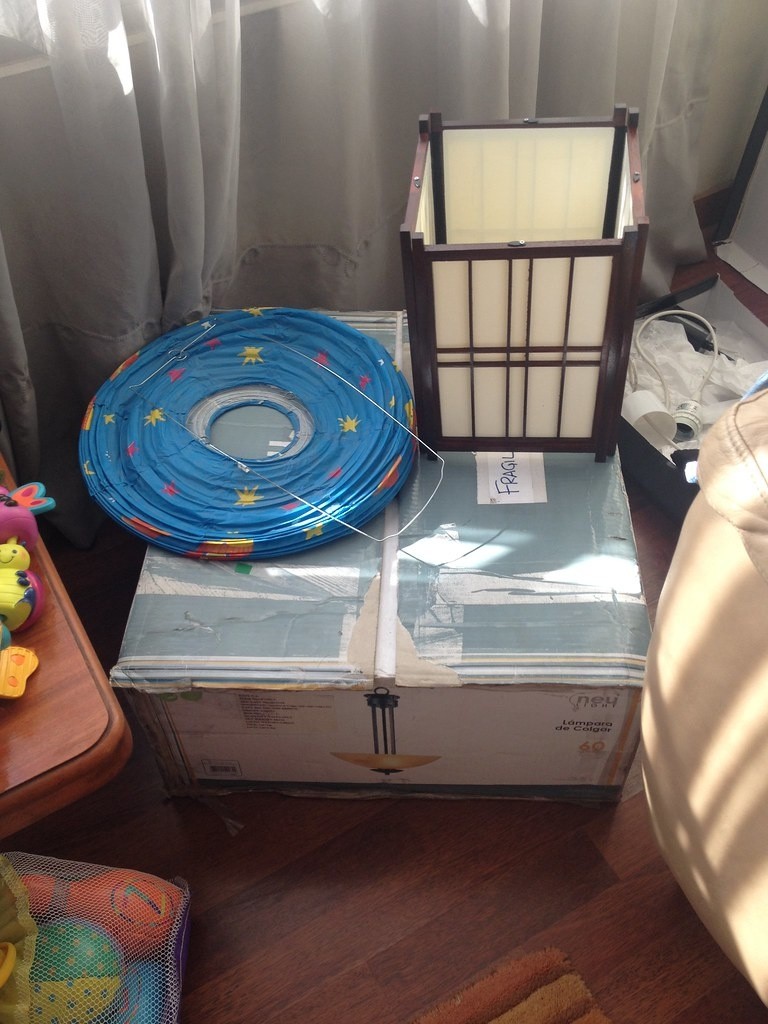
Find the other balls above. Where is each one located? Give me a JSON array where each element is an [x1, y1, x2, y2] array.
[[26, 917, 128, 1024]]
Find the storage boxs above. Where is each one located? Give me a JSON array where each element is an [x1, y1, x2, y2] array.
[[112, 313, 652, 804], [613, 272, 768, 530]]
